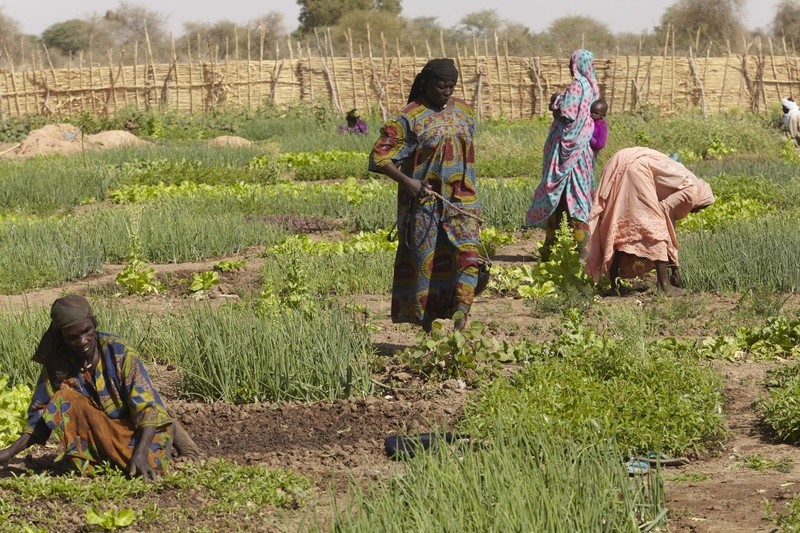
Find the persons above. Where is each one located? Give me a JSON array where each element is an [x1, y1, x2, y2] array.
[[0, 295, 195, 486], [526, 49, 716, 297], [782, 97, 800, 146], [369, 60, 481, 335], [337, 109, 367, 134]]
